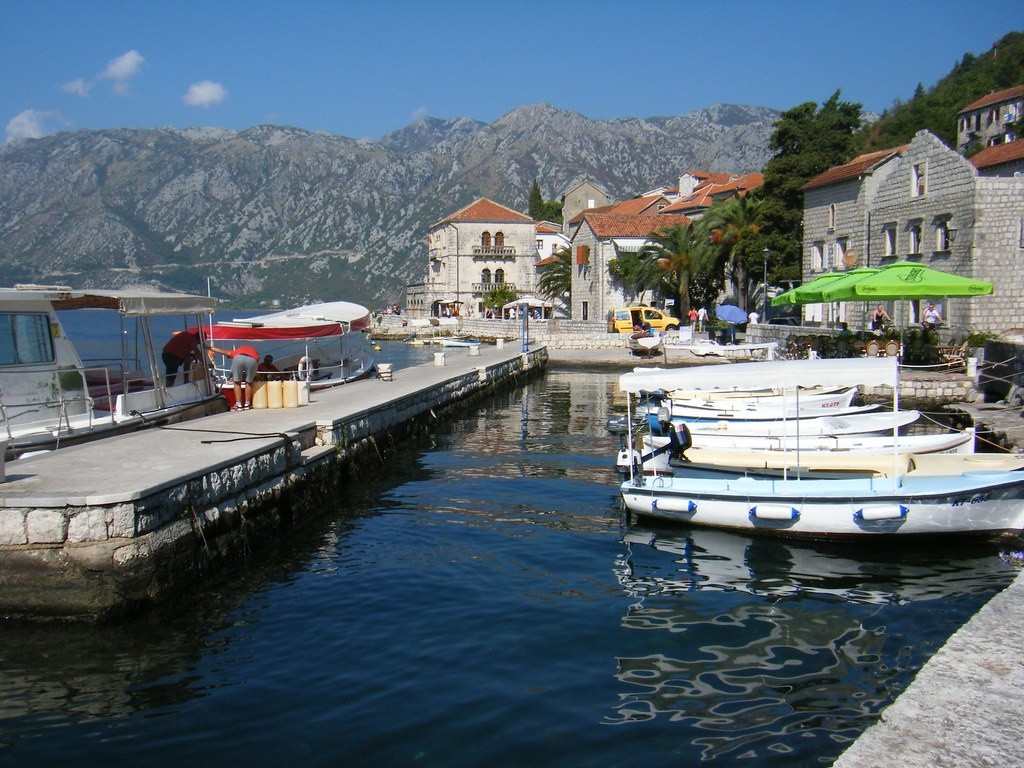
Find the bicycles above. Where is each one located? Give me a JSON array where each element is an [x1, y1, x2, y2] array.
[[926, 323, 944, 345], [875, 320, 892, 333]]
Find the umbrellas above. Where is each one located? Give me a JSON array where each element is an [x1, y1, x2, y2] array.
[[716, 305, 747, 342], [823, 258, 994, 367], [771, 272, 845, 337], [796, 266, 883, 340]]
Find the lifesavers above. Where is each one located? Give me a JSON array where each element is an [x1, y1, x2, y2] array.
[[298, 356, 314, 381]]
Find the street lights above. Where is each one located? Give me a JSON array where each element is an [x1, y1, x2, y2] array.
[[762, 246, 770, 324]]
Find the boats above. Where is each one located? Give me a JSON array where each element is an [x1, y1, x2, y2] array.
[[605, 354, 1024, 533], [173, 301, 373, 411], [0, 284, 226, 460], [627, 334, 661, 358], [406, 336, 482, 347]]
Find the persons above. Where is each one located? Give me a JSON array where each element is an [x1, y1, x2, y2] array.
[[922, 303, 944, 330], [258, 355, 285, 380], [664, 306, 670, 315], [632, 321, 651, 339], [749, 310, 759, 324], [204, 343, 259, 411], [443, 306, 459, 316], [874, 304, 890, 329], [839, 322, 851, 336], [384, 303, 400, 315], [486, 305, 499, 319], [509, 307, 541, 319], [698, 306, 709, 333], [161, 330, 207, 387], [688, 306, 698, 332]]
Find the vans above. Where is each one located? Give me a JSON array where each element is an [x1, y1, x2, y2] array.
[[608, 307, 682, 333]]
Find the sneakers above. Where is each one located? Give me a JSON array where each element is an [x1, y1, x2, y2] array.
[[244, 404, 250, 409], [230, 404, 243, 411]]
